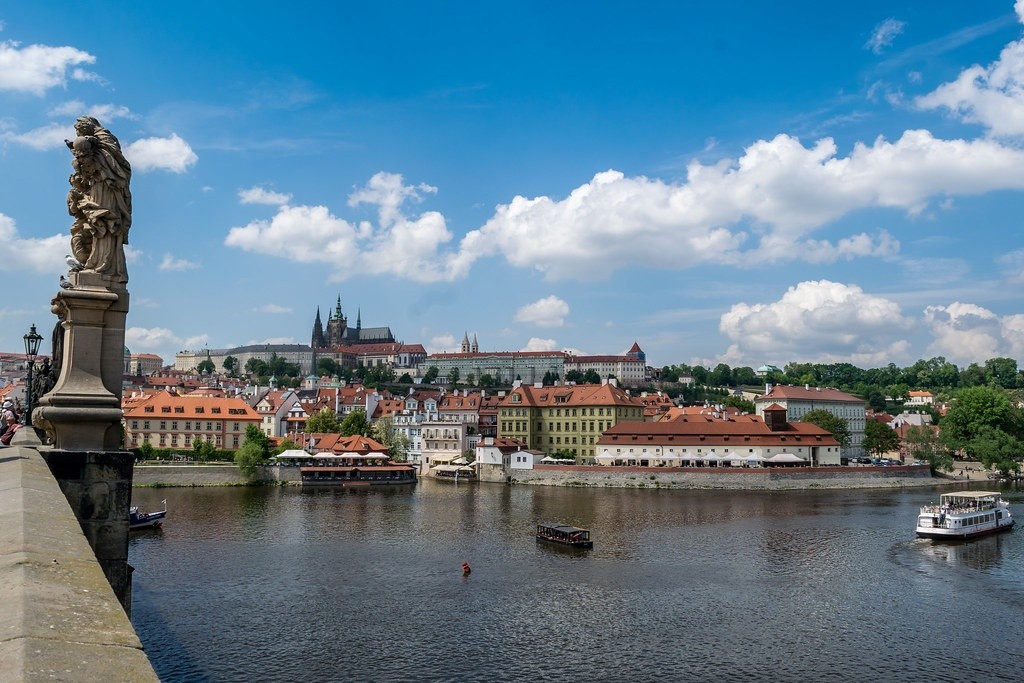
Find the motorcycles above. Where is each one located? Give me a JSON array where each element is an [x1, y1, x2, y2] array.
[[462, 562, 471, 573]]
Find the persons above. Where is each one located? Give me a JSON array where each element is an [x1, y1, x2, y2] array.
[[945, 499, 995, 508], [40, 358, 53, 375], [954, 474, 955, 479], [0, 397, 23, 445], [65, 117, 130, 274], [972, 468, 974, 473], [967, 474, 969, 479], [979, 466, 980, 471]]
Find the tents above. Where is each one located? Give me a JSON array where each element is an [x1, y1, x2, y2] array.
[[278, 450, 311, 465], [339, 452, 364, 458], [314, 452, 337, 458], [365, 453, 389, 459]]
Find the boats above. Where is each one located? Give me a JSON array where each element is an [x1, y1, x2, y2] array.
[[914, 491, 1016, 539], [536, 523, 593, 548], [130, 499, 167, 530]]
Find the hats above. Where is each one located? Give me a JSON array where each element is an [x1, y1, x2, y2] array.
[[1, 409, 7, 413], [3, 401, 13, 409], [3, 397, 16, 403]]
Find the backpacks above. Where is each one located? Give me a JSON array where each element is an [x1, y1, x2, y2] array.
[[1, 414, 7, 429]]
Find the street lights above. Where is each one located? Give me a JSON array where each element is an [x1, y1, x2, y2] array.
[[23, 323, 44, 426]]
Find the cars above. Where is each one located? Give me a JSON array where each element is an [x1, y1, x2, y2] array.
[[848, 457, 924, 467]]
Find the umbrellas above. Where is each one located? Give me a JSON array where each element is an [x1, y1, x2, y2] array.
[[640, 452, 657, 466], [617, 452, 635, 465], [680, 452, 701, 466], [723, 452, 744, 461], [746, 453, 766, 461], [659, 452, 679, 467], [704, 452, 721, 466], [596, 451, 614, 465]]
